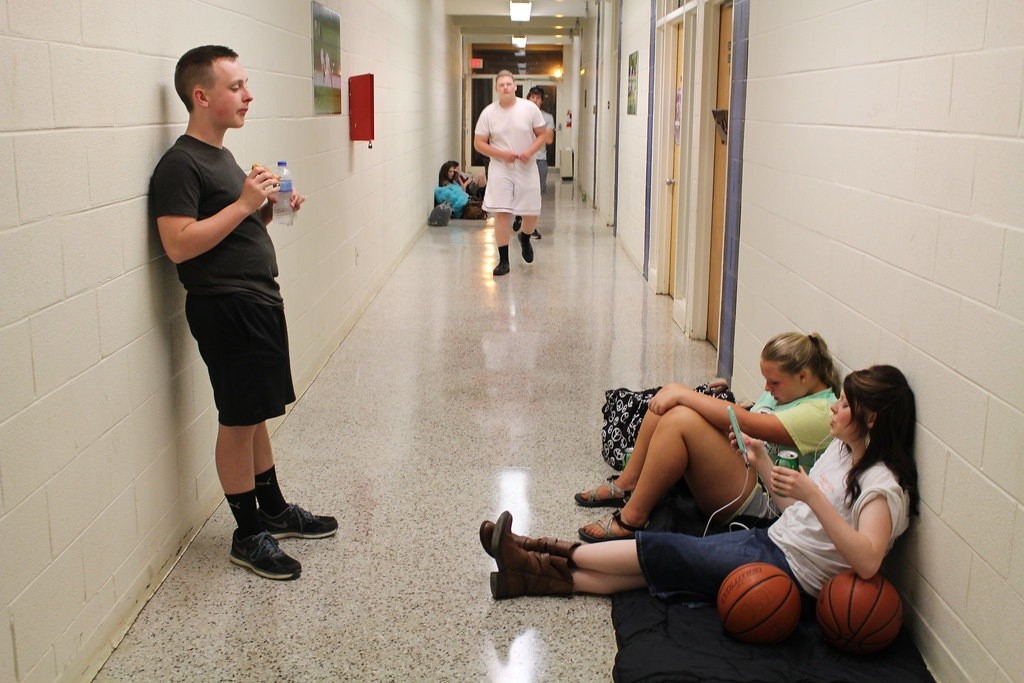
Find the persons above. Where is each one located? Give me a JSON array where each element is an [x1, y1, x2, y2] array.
[[439, 163, 488, 220], [513, 86, 555, 239], [447, 160, 486, 201], [479, 364, 921, 600], [574, 329, 841, 543], [474, 70, 548, 276], [149, 44, 338, 581]]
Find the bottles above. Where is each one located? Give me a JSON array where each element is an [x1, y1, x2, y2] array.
[[273, 161, 293, 225]]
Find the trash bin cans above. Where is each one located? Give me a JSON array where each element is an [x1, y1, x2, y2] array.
[[560, 148, 574, 181]]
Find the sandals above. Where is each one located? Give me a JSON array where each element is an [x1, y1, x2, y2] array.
[[578, 510, 650, 543], [574, 477, 632, 506]]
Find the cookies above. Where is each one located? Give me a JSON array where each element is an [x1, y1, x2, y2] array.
[[252, 163, 280, 181]]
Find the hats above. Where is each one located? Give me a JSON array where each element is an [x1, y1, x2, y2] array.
[[527, 88, 544, 100]]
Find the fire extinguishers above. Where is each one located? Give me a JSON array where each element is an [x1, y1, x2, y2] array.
[[566, 110, 572, 127]]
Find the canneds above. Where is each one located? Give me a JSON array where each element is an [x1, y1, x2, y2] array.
[[775, 450, 798, 497]]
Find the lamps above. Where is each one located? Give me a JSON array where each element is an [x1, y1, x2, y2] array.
[[510, 0, 532, 23], [512, 35, 527, 49]]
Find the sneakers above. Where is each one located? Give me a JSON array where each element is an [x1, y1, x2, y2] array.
[[257, 502, 338, 539], [532, 230, 541, 238], [494, 263, 509, 275], [513, 216, 522, 231], [230, 528, 302, 579], [518, 232, 533, 263]]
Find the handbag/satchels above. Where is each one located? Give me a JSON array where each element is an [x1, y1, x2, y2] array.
[[467, 196, 487, 220], [428, 198, 452, 225], [601, 384, 736, 471]]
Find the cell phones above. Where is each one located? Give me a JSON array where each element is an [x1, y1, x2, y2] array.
[[728, 406, 748, 454]]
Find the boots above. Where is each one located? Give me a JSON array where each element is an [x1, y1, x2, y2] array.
[[490, 511, 573, 600], [479, 520, 581, 558]]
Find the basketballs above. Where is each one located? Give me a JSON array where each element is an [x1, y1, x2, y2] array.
[[716, 562, 801, 645], [816, 568, 903, 655]]
